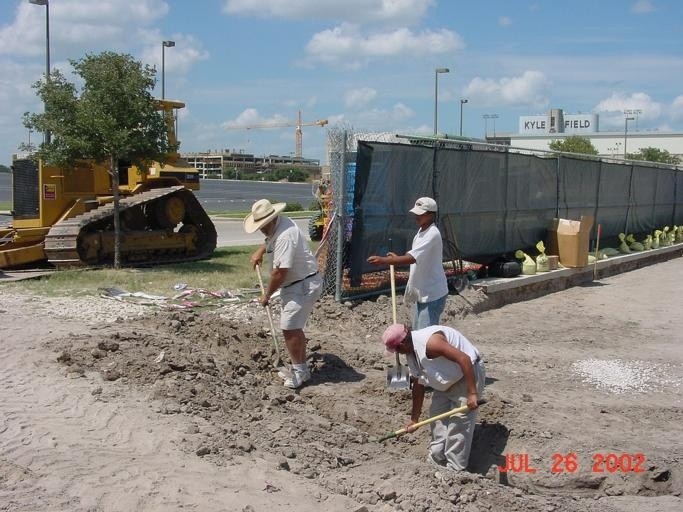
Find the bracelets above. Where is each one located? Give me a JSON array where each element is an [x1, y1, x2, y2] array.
[[263, 290, 273, 297]]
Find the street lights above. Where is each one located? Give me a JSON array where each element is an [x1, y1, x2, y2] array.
[[480, 113, 498, 139], [624, 109, 642, 160], [434, 68, 449, 135], [460, 100, 467, 136], [162, 41, 175, 136], [29, 0, 50, 144]]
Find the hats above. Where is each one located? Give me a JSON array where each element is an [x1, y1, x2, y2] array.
[[407, 197, 438, 217], [381, 323, 410, 350], [242, 198, 286, 235]]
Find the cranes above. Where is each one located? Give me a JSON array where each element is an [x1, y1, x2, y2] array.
[[227, 109, 328, 160]]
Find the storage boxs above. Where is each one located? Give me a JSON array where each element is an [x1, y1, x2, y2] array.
[[546, 216, 594, 270]]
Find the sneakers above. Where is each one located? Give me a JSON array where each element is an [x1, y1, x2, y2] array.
[[277, 365, 312, 390]]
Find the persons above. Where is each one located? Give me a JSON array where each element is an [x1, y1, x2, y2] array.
[[242, 197, 325, 389], [365, 195, 449, 395], [380, 322, 487, 471]]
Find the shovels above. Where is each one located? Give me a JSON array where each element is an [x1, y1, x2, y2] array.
[[387, 240, 410, 392], [254, 263, 297, 380]]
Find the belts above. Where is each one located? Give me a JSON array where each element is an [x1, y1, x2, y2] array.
[[282, 269, 320, 289]]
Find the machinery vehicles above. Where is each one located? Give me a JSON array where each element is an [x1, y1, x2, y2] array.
[[0, 100, 216, 269], [309, 173, 332, 240]]
